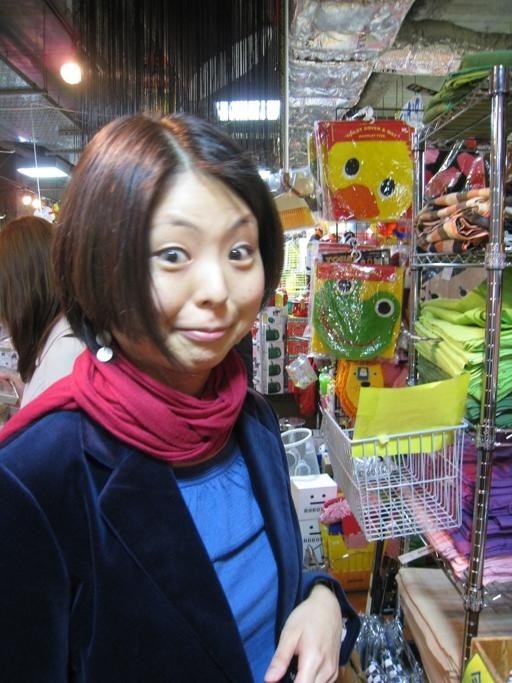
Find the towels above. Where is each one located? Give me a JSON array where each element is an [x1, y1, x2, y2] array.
[[391, 46, 512, 683]]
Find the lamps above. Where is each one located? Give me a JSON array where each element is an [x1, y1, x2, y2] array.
[[14, 153, 71, 181]]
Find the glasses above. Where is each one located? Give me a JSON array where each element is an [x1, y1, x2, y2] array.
[[285, 449, 311, 476]]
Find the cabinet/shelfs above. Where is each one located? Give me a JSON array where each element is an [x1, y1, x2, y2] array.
[[383, 63, 512, 683]]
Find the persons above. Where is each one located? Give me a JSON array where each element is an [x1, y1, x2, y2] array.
[[34, 198, 55, 224], [0, 214, 91, 412], [0, 111, 363, 683], [49, 198, 61, 220]]
